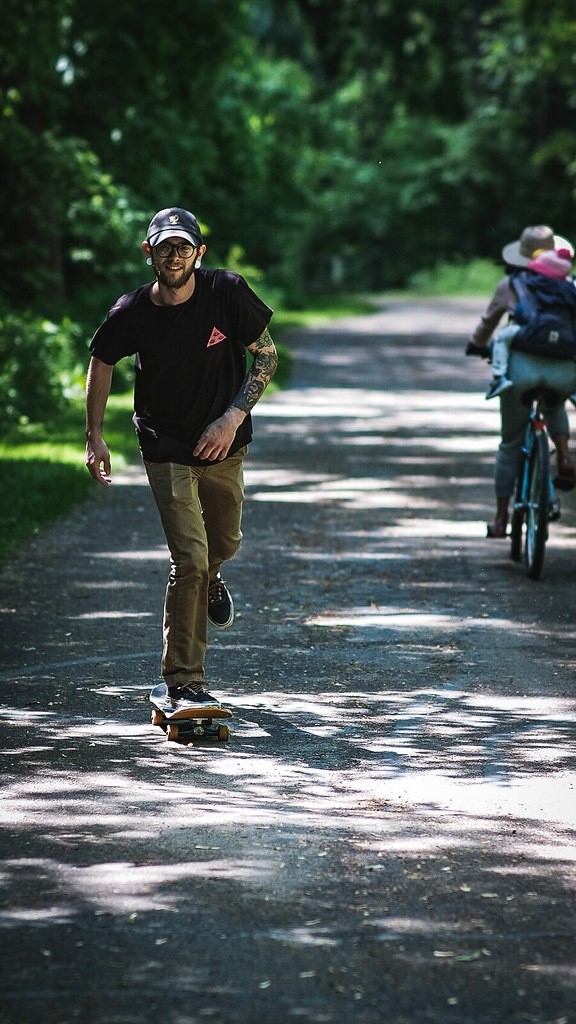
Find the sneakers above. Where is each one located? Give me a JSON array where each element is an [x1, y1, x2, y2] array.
[[207, 571, 234, 630], [164, 682, 221, 710]]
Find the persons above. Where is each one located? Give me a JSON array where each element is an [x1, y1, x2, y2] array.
[[85, 208, 278, 709], [468, 224, 575, 537]]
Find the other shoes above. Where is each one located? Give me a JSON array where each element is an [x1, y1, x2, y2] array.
[[553, 464, 574, 490], [487, 519, 506, 538], [569, 390, 576, 406], [485, 374, 514, 399]]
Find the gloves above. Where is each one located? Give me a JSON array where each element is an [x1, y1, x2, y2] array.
[[466, 342, 483, 356]]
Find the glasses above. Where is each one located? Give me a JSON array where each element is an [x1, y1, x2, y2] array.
[[156, 243, 200, 259]]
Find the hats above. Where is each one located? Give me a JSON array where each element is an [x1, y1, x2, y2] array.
[[146, 207, 202, 247], [503, 226, 574, 267], [529, 247, 573, 281]]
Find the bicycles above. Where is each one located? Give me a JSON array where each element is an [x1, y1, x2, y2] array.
[[484, 343, 559, 580]]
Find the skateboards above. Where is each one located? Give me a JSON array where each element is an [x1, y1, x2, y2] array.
[[149, 681, 236, 743]]
